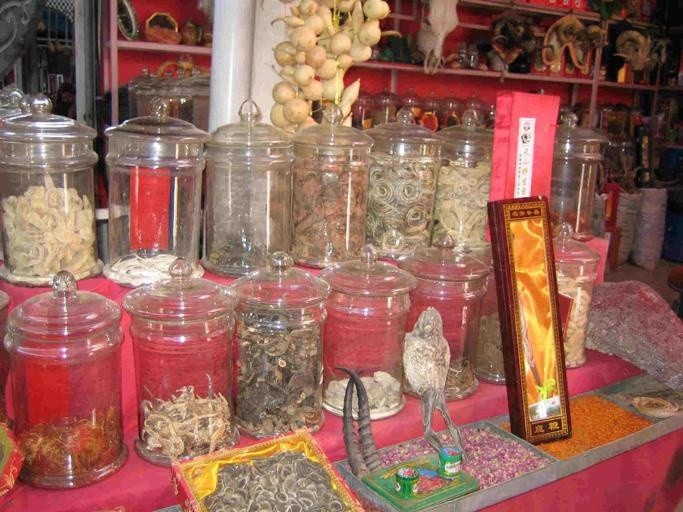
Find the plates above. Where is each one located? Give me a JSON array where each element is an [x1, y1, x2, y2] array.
[[615, 32, 647, 71]]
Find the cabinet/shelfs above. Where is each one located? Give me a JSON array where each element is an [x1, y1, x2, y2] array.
[[93, 1, 682, 267]]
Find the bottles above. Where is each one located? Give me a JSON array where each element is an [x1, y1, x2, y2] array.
[[291, 104, 374, 269], [202, 98, 294, 276], [227, 253, 329, 439], [428, 110, 494, 248], [314, 248, 408, 423], [101, 98, 207, 290], [397, 236, 482, 404], [3, 271, 133, 490], [359, 108, 439, 260], [469, 239, 508, 387], [550, 110, 604, 235], [1, 85, 102, 280], [540, 221, 600, 366], [351, 84, 601, 121], [120, 258, 239, 468]]
